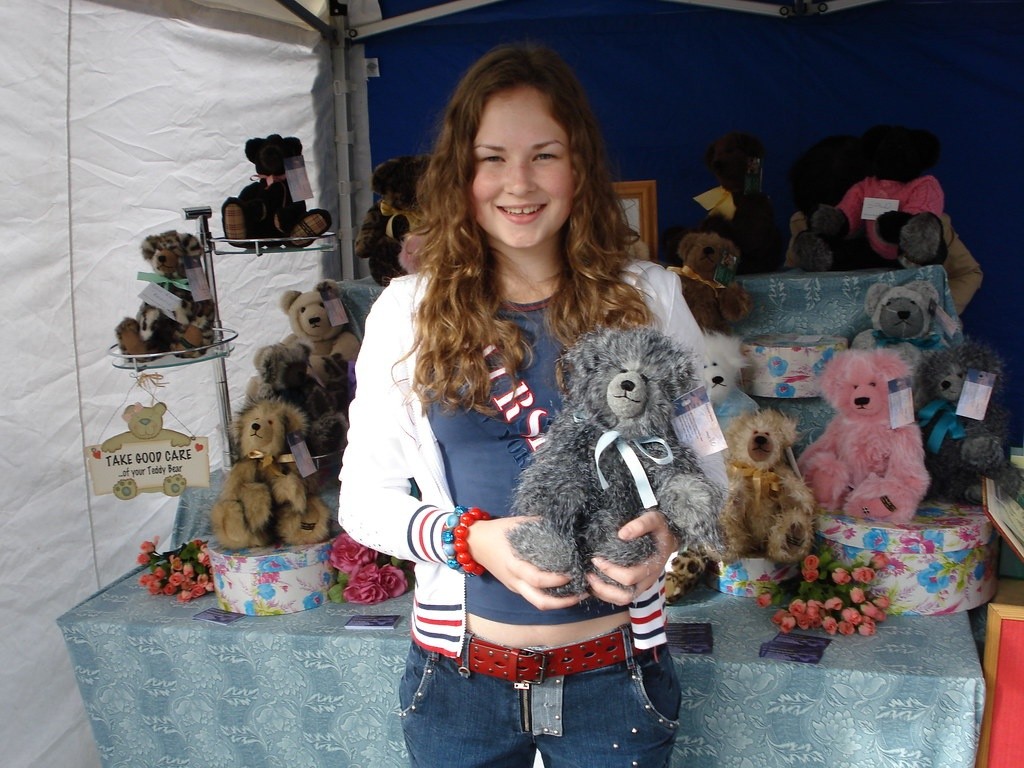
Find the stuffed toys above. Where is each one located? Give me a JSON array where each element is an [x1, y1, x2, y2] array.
[[510, 328, 723, 598], [721, 410, 814, 564], [797, 348, 929, 521], [919, 345, 1021, 503], [786, 175, 982, 315], [355, 154, 430, 285], [664, 548, 707, 596], [221, 134, 332, 247], [704, 330, 740, 403], [280, 279, 358, 387], [114, 230, 215, 362], [854, 280, 938, 394], [679, 133, 763, 334], [213, 343, 345, 548]]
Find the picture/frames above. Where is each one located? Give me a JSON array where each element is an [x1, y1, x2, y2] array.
[[610, 180, 658, 260]]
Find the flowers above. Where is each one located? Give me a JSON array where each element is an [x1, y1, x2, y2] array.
[[327, 533, 415, 606], [755, 550, 891, 637], [136, 539, 215, 603]]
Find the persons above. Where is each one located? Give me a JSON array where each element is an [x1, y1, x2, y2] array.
[[337, 48, 728, 768]]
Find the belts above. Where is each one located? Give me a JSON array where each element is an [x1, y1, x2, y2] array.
[[453, 626, 643, 684]]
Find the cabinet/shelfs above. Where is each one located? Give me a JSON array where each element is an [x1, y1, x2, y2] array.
[[54, 262, 985, 768]]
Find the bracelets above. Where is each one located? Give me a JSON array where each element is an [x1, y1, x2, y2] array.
[[443, 507, 490, 576]]
